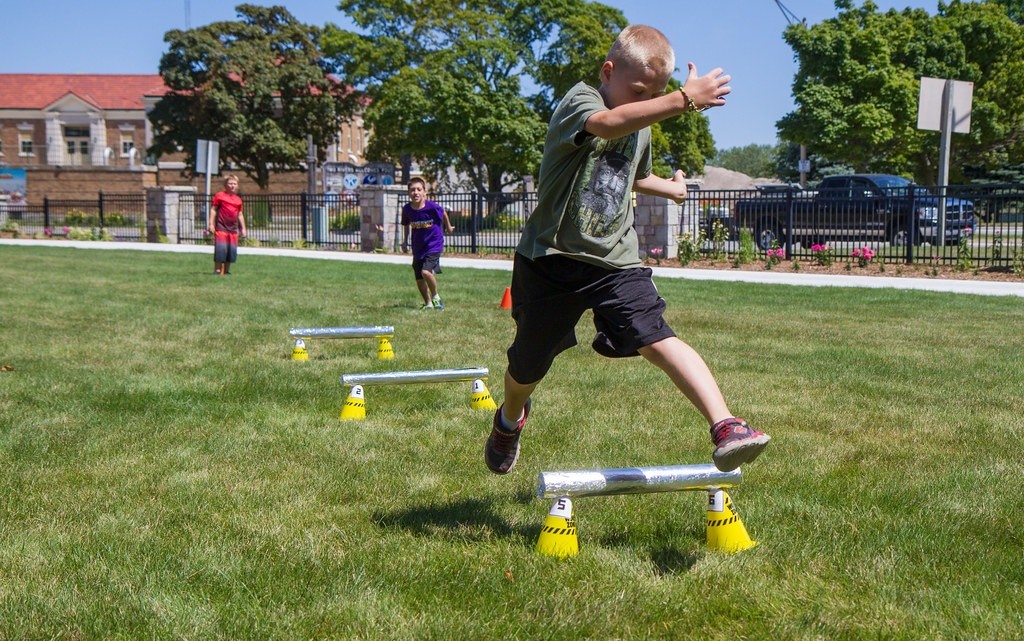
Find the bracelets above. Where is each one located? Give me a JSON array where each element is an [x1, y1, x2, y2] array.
[[680, 86, 710, 112]]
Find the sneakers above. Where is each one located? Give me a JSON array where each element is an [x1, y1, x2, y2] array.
[[485, 398, 532, 475], [710, 418, 771, 472]]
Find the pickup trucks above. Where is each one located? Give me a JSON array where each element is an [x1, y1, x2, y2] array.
[[734, 174, 971, 250]]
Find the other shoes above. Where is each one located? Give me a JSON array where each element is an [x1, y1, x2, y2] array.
[[432, 299, 444, 312], [420, 304, 433, 311]]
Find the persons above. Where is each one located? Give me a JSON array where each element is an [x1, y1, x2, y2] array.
[[401, 177, 455, 312], [209, 173, 246, 274], [483, 24, 771, 476]]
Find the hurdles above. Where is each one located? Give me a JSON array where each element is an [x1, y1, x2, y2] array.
[[529, 456, 762, 570], [285, 322, 399, 366], [333, 363, 503, 426]]
